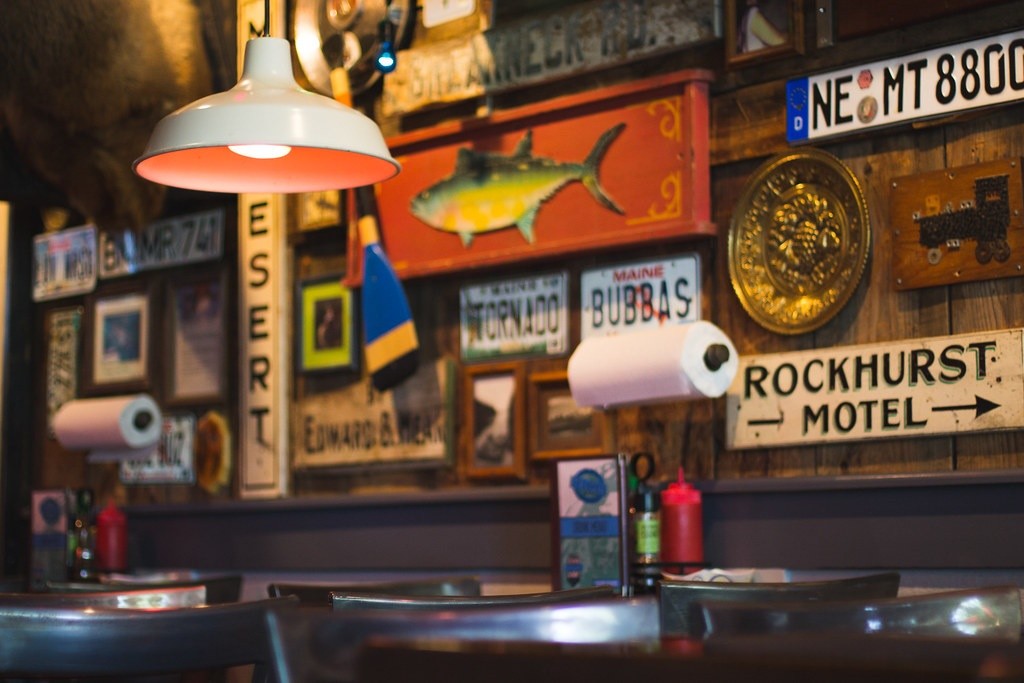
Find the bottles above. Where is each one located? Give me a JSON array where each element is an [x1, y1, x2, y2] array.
[[96, 497, 127, 568], [660, 465, 702, 573], [632, 495, 662, 578]]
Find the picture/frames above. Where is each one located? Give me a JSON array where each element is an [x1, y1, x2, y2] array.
[[528, 372, 616, 460], [297, 270, 363, 377], [724, 1, 806, 69], [80, 280, 158, 394], [460, 359, 530, 484]]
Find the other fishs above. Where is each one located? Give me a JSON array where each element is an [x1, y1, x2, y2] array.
[[411, 123, 625, 247]]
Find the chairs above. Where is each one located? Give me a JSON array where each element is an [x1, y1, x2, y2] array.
[[0, 563, 1024, 683]]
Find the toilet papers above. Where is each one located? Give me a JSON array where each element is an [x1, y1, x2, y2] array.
[[49, 396, 161, 447], [568, 320, 739, 407]]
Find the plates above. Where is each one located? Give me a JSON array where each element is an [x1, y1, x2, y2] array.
[[725, 143, 872, 337]]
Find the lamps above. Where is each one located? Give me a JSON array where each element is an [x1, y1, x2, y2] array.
[[132, 1, 400, 193]]
